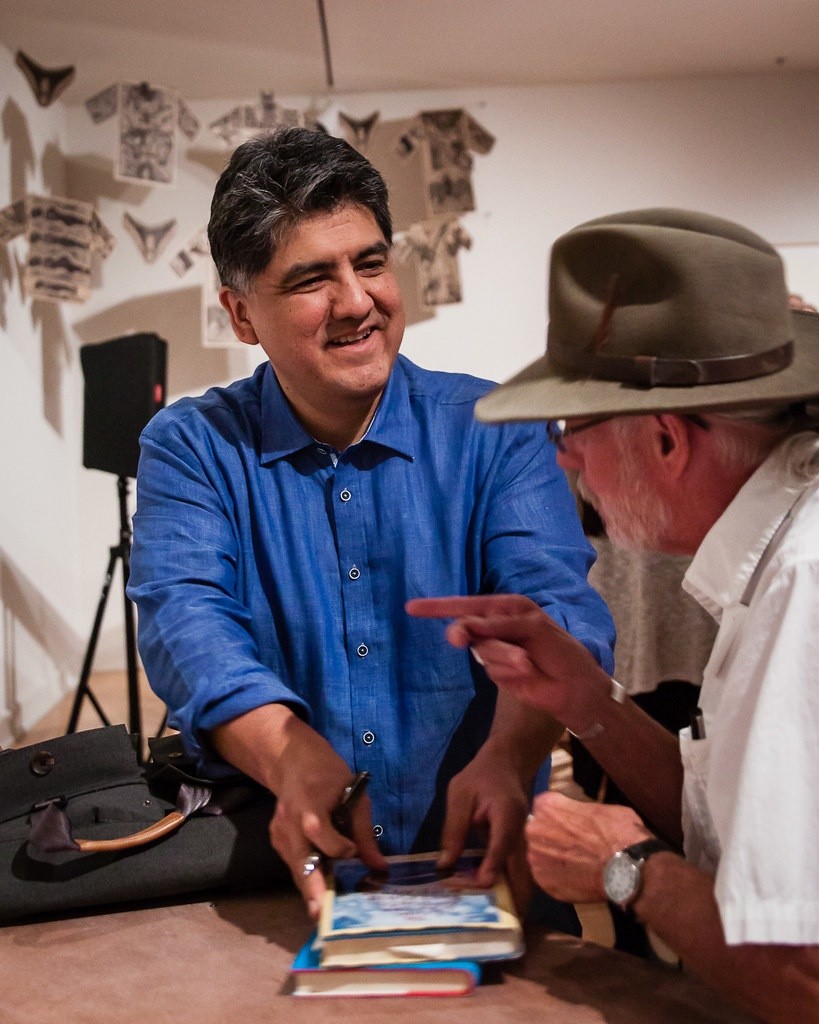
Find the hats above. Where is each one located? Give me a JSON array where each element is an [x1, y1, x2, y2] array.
[[472, 210, 819, 423]]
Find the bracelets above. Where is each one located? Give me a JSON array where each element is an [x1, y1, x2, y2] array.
[[566, 679, 626, 740]]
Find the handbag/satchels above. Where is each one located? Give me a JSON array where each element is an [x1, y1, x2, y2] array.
[[0, 729, 294, 922]]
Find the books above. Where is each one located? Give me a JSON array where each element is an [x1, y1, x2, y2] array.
[[291, 847, 528, 997]]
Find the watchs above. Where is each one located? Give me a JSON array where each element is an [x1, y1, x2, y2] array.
[[602, 837, 686, 922]]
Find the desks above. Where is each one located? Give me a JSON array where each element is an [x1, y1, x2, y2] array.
[[0, 897, 733, 1024]]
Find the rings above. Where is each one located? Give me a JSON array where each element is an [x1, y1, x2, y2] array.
[[299, 852, 320, 876], [469, 645, 486, 667]]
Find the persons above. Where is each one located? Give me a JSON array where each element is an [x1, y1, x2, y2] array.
[[118, 126, 617, 919], [399, 204, 819, 1024]]
